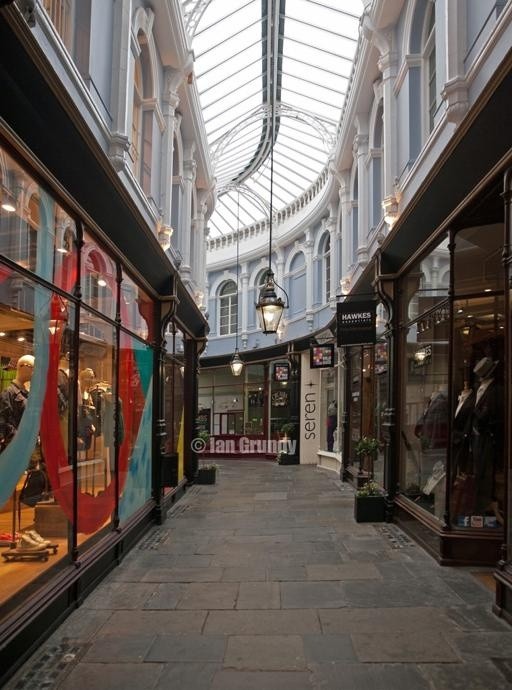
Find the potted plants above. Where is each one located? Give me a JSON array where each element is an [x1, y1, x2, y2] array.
[[276, 422, 299, 464], [355, 402, 387, 523], [197, 413, 216, 483]]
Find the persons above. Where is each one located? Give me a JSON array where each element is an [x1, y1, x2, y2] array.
[[326, 400, 337, 451], [0, 353, 125, 505], [414, 354, 506, 526]]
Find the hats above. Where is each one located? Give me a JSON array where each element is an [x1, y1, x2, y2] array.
[[473, 356, 500, 383]]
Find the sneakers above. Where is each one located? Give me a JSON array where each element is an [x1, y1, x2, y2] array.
[[25, 530, 51, 548], [16, 535, 47, 551]]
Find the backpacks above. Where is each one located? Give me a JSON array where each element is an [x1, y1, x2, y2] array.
[[19, 469, 50, 508]]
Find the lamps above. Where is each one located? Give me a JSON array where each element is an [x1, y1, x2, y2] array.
[[257, 89, 285, 336], [2, 164, 24, 214], [228, 185, 247, 377], [58, 213, 74, 254], [38, 204, 70, 339], [123, 282, 137, 308]]
